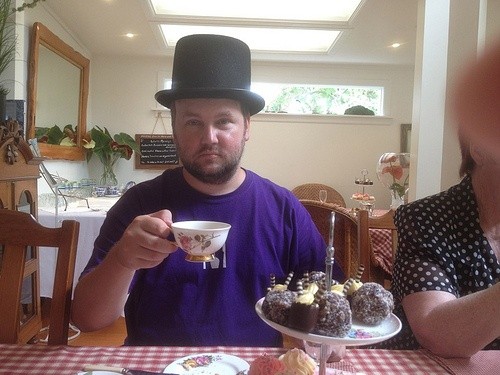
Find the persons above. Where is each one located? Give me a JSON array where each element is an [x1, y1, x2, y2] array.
[[360, 40, 500, 358], [71, 34, 346, 348]]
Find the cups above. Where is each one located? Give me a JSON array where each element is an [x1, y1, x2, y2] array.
[[96, 185, 118, 197], [166, 220, 231, 262]]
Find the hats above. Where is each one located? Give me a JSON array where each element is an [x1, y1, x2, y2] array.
[[154, 33, 265, 117]]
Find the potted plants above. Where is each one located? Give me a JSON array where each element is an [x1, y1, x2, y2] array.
[[0, 0, 47, 123]]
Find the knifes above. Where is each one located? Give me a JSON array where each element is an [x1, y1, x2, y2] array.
[[82, 364, 179, 375]]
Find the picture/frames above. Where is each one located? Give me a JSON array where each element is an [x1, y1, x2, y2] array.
[[400, 124, 411, 168]]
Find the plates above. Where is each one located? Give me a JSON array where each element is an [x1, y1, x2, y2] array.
[[78, 371, 122, 375], [163, 353, 250, 375]]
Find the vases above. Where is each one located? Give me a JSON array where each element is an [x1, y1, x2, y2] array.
[[100, 154, 121, 196]]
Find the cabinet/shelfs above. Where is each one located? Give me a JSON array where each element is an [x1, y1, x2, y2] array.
[[0, 117, 45, 345]]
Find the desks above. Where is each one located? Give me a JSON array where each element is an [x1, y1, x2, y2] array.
[[340, 207, 393, 279], [0, 341, 500, 374], [38, 195, 125, 301]]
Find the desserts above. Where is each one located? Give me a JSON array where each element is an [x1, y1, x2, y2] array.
[[352, 192, 375, 200], [351, 207, 360, 211], [263, 264, 394, 338], [354, 178, 374, 185], [247, 348, 317, 375]]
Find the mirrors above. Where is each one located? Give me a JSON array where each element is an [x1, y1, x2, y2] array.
[[29, 21, 92, 162]]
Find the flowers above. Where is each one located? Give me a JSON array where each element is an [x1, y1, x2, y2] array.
[[34, 122, 78, 146], [81, 124, 142, 186]]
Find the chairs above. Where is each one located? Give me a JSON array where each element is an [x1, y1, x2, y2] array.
[[299, 199, 376, 281], [0, 207, 80, 347], [291, 184, 345, 209], [355, 209, 396, 292]]
